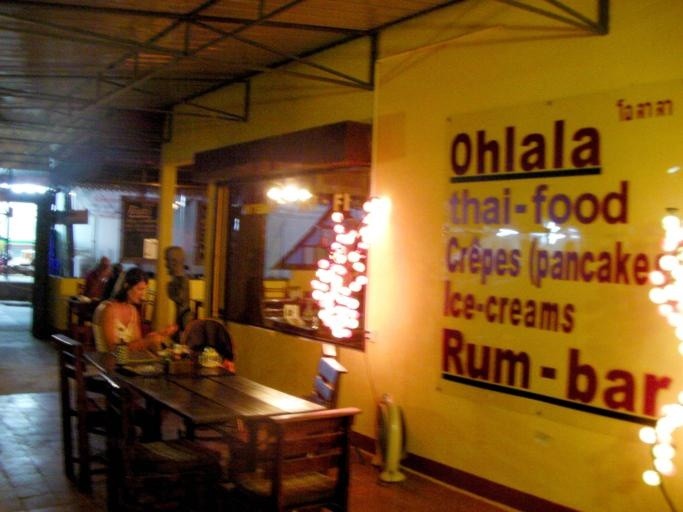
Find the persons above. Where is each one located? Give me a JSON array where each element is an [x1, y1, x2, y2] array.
[[80, 256, 114, 311], [90, 267, 180, 443]]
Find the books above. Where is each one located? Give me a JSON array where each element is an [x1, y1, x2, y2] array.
[[113, 348, 158, 363]]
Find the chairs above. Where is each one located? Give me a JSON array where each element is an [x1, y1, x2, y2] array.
[[179, 319, 236, 368], [101, 374, 223, 511], [52, 332, 149, 483], [232, 406, 361, 511], [299, 357, 348, 410]]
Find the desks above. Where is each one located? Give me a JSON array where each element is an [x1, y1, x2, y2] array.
[[83, 338, 327, 473]]
